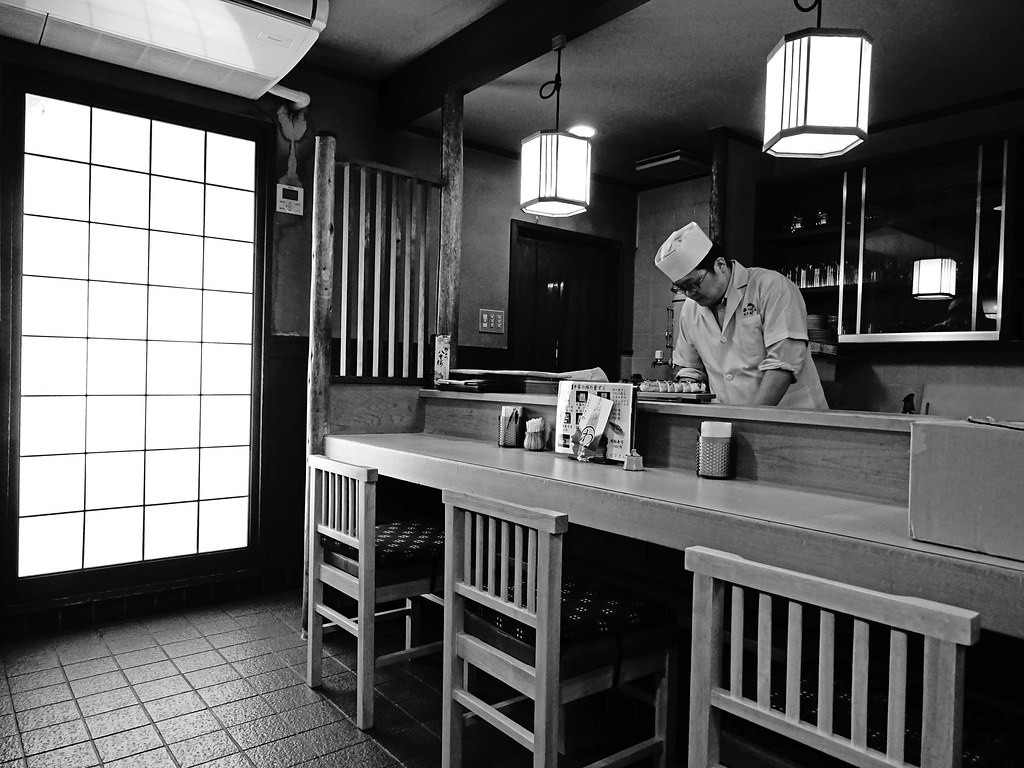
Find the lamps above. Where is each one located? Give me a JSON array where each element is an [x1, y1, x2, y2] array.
[[761, 0, 875, 160], [911, 220, 959, 303], [516, 33, 594, 220]]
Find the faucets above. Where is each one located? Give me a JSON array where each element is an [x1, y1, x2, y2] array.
[[649, 348, 672, 371]]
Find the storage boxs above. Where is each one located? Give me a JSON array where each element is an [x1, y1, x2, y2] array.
[[902, 417, 1024, 563]]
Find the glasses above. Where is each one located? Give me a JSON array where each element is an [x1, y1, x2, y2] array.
[[670, 268, 712, 294]]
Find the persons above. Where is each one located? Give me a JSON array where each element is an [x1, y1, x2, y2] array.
[[654, 221, 830, 412]]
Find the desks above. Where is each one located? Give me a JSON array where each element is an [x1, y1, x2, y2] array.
[[322, 426, 1023, 644]]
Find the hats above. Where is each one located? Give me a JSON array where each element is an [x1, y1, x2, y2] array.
[[655, 221, 714, 282]]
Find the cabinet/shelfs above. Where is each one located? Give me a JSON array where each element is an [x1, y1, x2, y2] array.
[[749, 135, 1017, 355]]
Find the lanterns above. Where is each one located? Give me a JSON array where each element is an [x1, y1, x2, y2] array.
[[761, 26, 873, 160], [911, 256, 958, 302], [521, 130, 593, 218]]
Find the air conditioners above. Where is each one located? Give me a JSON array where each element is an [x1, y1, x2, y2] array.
[[1, 0, 332, 103]]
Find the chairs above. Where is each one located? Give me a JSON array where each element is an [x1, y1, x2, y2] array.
[[301, 452, 481, 734], [679, 545, 982, 767], [438, 487, 684, 768]]
